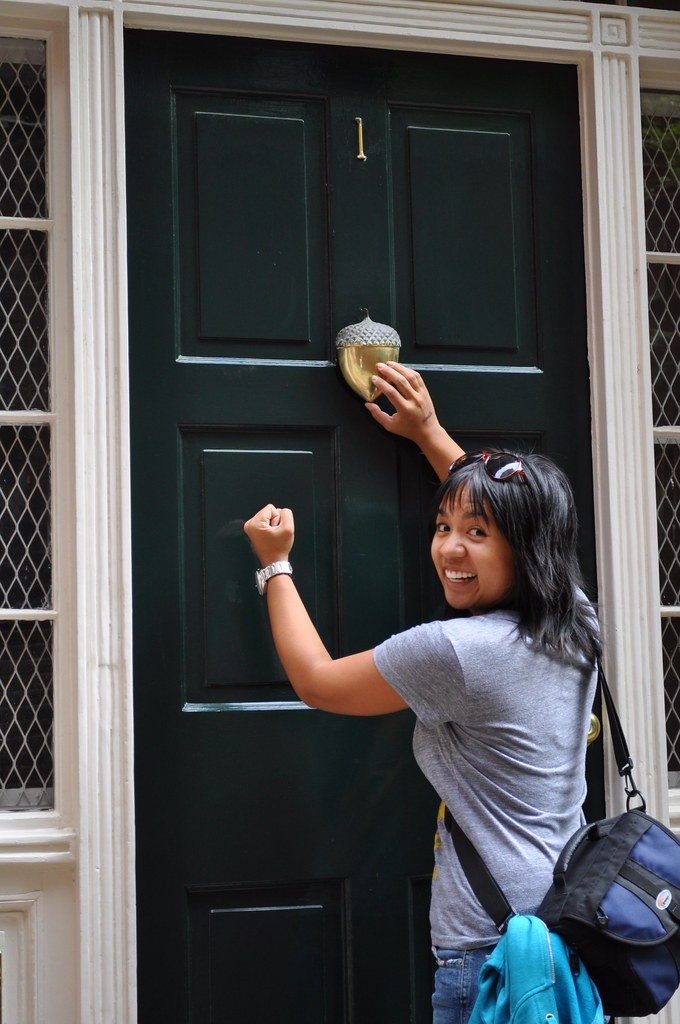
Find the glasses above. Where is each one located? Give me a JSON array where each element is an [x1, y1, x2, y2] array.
[[448, 448, 533, 496]]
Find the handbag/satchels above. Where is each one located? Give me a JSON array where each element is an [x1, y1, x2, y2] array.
[[535, 808, 680, 1017]]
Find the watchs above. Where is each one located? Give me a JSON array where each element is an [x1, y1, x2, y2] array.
[[255, 561, 293, 595]]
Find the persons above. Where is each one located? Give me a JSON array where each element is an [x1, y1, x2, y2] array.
[[243, 360, 602, 1024]]
